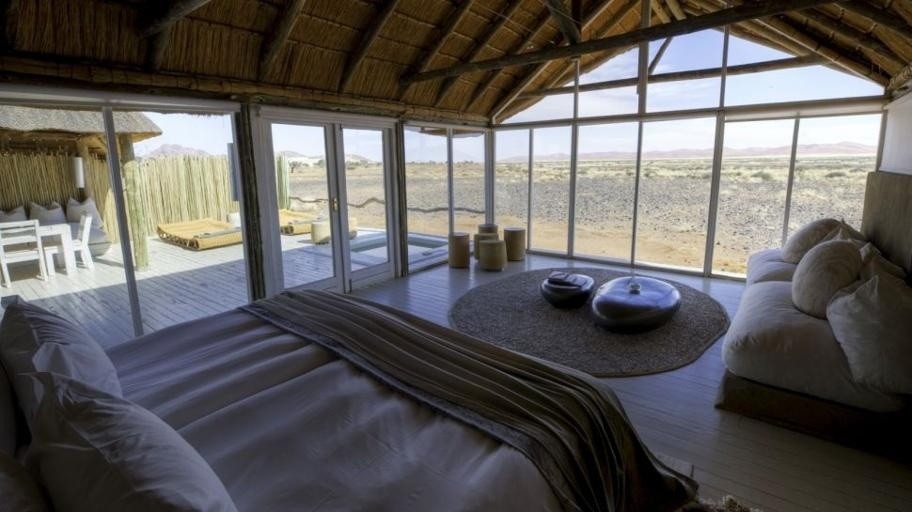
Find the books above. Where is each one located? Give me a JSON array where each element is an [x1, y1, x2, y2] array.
[[549, 270, 587, 286]]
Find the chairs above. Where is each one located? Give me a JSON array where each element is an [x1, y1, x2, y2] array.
[[0, 213, 97, 283]]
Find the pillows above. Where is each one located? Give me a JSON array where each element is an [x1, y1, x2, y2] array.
[[0, 294, 123, 442], [11, 372, 234, 511], [780, 217, 863, 316]]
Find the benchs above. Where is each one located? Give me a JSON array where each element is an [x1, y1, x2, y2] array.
[[713, 245, 912, 462]]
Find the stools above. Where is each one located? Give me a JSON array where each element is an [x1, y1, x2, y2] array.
[[309, 219, 329, 245], [447, 222, 527, 270], [347, 218, 357, 239]]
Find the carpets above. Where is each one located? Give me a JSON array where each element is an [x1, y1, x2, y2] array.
[[449, 266, 731, 381]]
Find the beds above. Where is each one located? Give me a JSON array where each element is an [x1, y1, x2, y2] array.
[[155, 215, 242, 253], [0, 284, 629, 511], [276, 207, 315, 236]]
